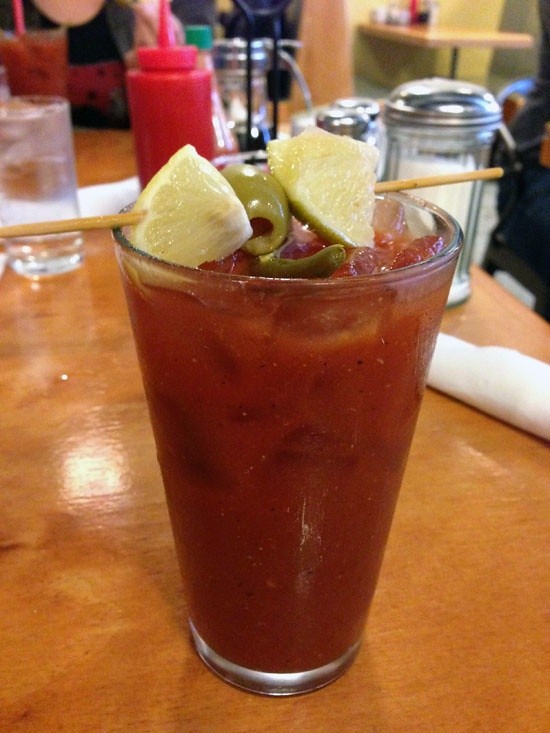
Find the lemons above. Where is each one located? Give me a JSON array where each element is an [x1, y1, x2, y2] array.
[[263, 123, 381, 255], [118, 146, 252, 277]]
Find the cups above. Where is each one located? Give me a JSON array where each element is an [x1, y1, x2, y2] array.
[[1, 97, 88, 281], [0, 19, 70, 108], [113, 162, 467, 698]]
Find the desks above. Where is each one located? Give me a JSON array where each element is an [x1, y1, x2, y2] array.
[[357, 22, 535, 79]]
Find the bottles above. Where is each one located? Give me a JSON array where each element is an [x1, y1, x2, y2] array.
[[118, 4, 521, 307]]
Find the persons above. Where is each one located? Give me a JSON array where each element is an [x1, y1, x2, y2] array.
[[0, 0, 188, 132], [495, 1, 549, 292]]
